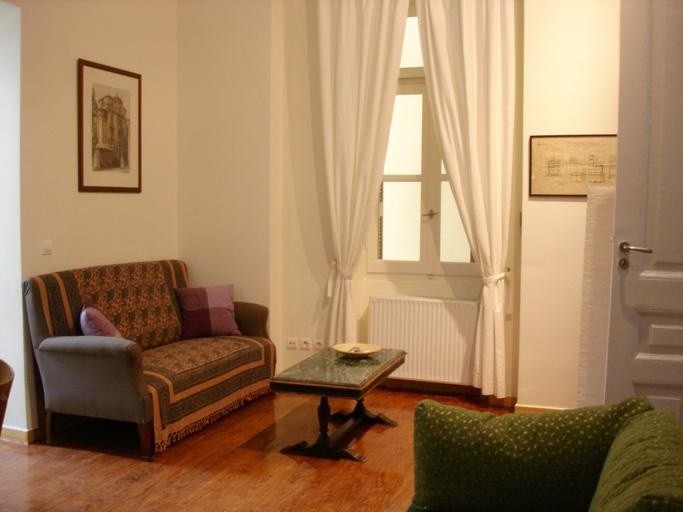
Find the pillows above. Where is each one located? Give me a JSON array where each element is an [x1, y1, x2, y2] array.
[[173, 284, 243, 339], [79, 302, 124, 337]]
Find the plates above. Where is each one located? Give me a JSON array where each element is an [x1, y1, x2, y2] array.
[[333, 343, 380, 359]]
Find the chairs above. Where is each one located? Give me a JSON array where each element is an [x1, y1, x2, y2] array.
[[407, 392, 683, 512]]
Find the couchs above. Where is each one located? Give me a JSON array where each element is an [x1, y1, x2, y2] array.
[[23, 259, 277, 461]]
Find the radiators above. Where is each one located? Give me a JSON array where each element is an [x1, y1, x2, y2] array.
[[366, 295, 480, 389]]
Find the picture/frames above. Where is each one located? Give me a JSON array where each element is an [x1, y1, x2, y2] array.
[[527, 134, 618, 198], [76, 56, 142, 195]]
